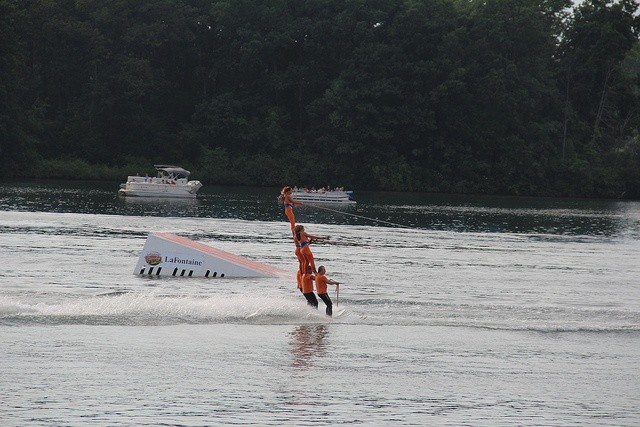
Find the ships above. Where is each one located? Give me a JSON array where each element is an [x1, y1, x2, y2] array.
[[289, 191, 357, 204], [118, 164, 203, 198]]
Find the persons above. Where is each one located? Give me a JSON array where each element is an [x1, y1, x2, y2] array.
[[316, 266, 339, 317], [294, 185, 344, 193], [297, 266, 302, 294], [278, 186, 303, 236], [297, 225, 320, 277], [162, 179, 175, 184], [293, 226, 304, 275], [302, 266, 317, 309]]
